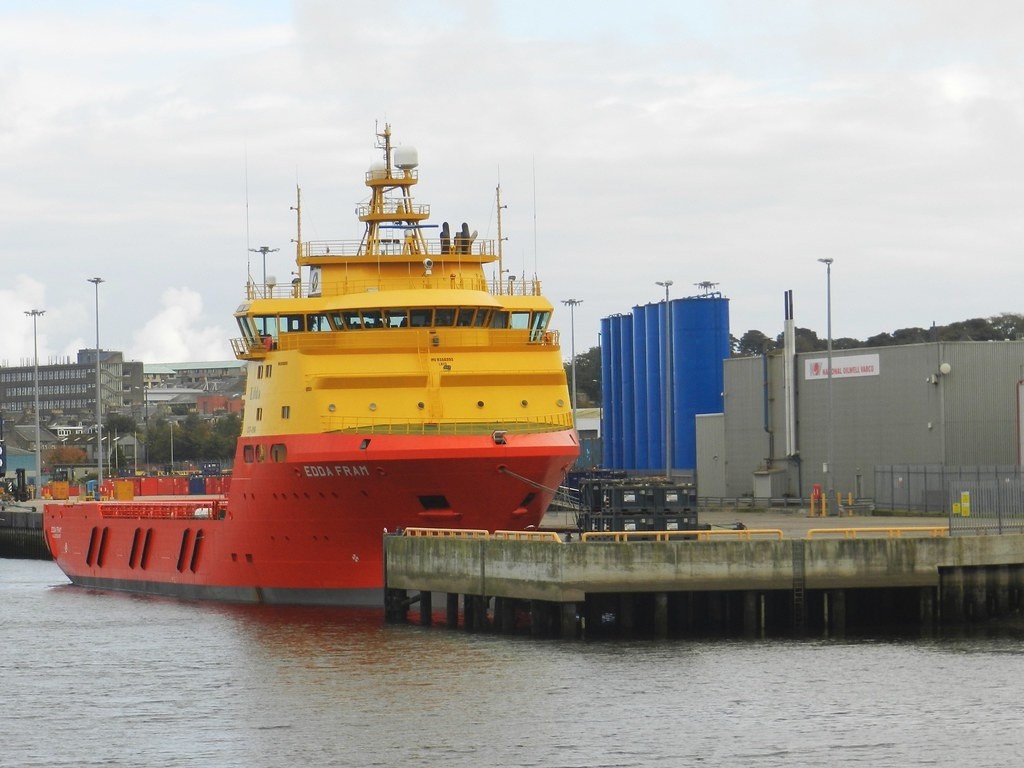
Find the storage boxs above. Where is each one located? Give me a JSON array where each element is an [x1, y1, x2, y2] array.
[[547, 470, 698, 541], [103, 463, 232, 497]]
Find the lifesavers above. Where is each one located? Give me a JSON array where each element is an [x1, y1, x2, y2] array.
[[266, 336, 272, 351]]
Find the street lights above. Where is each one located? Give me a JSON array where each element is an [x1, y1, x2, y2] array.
[[817, 257, 835, 515], [597, 332, 602, 469], [23, 308, 49, 500], [87, 277, 105, 488], [655, 280, 677, 482], [143, 386, 151, 478], [167, 420, 174, 471], [559, 298, 584, 436], [248, 245, 281, 335], [694, 280, 719, 299]]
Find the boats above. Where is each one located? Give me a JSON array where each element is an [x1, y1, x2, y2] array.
[[40, 116, 582, 611]]
